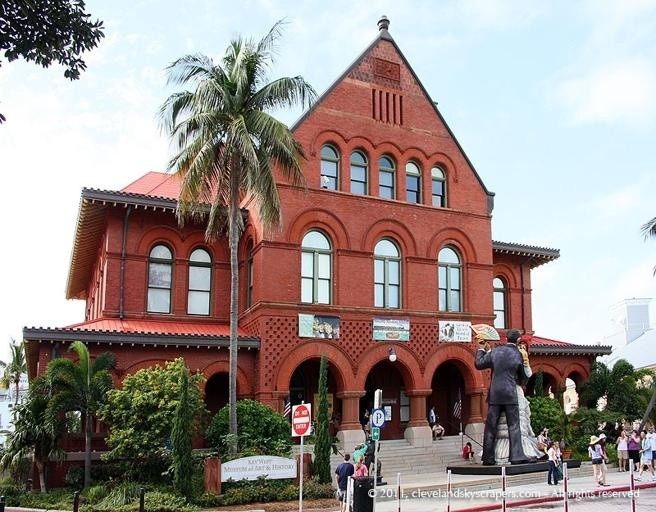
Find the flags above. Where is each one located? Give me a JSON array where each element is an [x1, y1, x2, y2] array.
[[453, 399, 461, 419], [284, 397, 292, 417]]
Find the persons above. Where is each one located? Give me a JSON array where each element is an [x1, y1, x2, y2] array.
[[334, 405, 474, 512], [474, 328, 545, 465], [315, 324, 328, 338], [536, 427, 656, 486]]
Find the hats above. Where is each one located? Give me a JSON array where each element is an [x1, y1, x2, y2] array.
[[589, 435, 600, 445], [599, 434, 607, 440]]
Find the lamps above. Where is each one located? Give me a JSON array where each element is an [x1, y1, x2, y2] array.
[[387, 345, 397, 362]]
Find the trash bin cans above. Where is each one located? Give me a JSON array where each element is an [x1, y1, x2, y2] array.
[[349, 476, 374, 512]]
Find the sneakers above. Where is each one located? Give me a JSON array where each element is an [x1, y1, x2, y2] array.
[[597, 480, 612, 487], [617, 467, 656, 482]]
